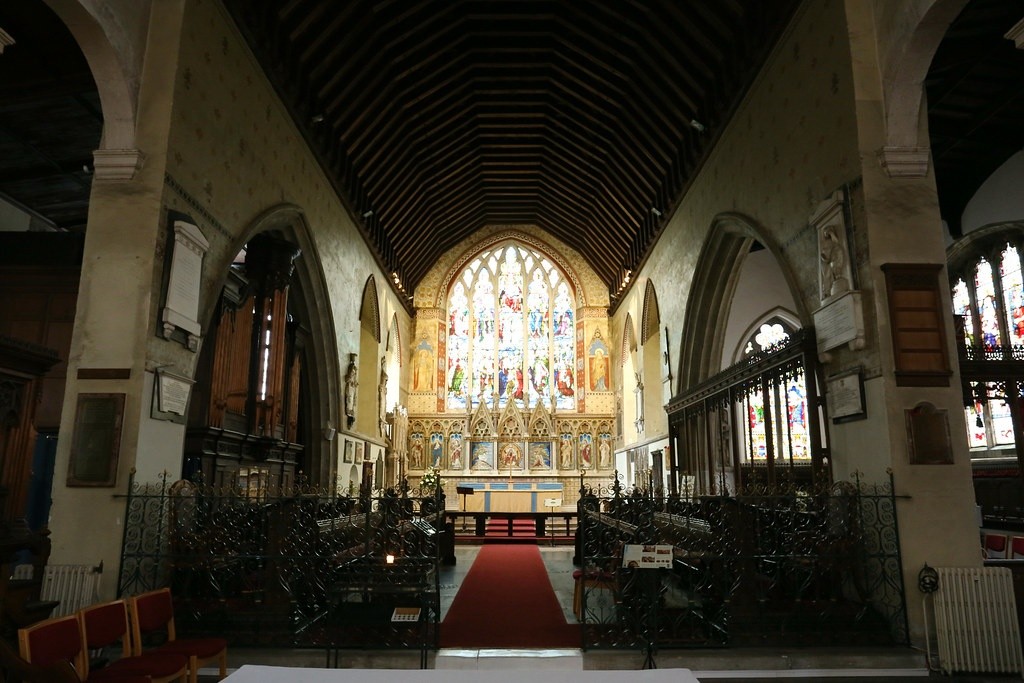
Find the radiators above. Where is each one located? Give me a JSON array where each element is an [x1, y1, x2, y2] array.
[[932, 567, 1024, 676]]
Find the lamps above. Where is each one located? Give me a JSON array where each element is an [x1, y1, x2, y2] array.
[[311, 114, 324, 124], [690, 119, 707, 134], [651, 206, 662, 216], [363, 210, 374, 218]]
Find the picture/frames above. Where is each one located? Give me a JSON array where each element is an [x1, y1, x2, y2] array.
[[66, 392, 126, 487]]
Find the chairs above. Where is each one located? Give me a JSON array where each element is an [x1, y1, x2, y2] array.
[[985, 534, 1009, 559], [17, 587, 227, 683], [1011, 536, 1024, 560], [573, 540, 625, 622]]
